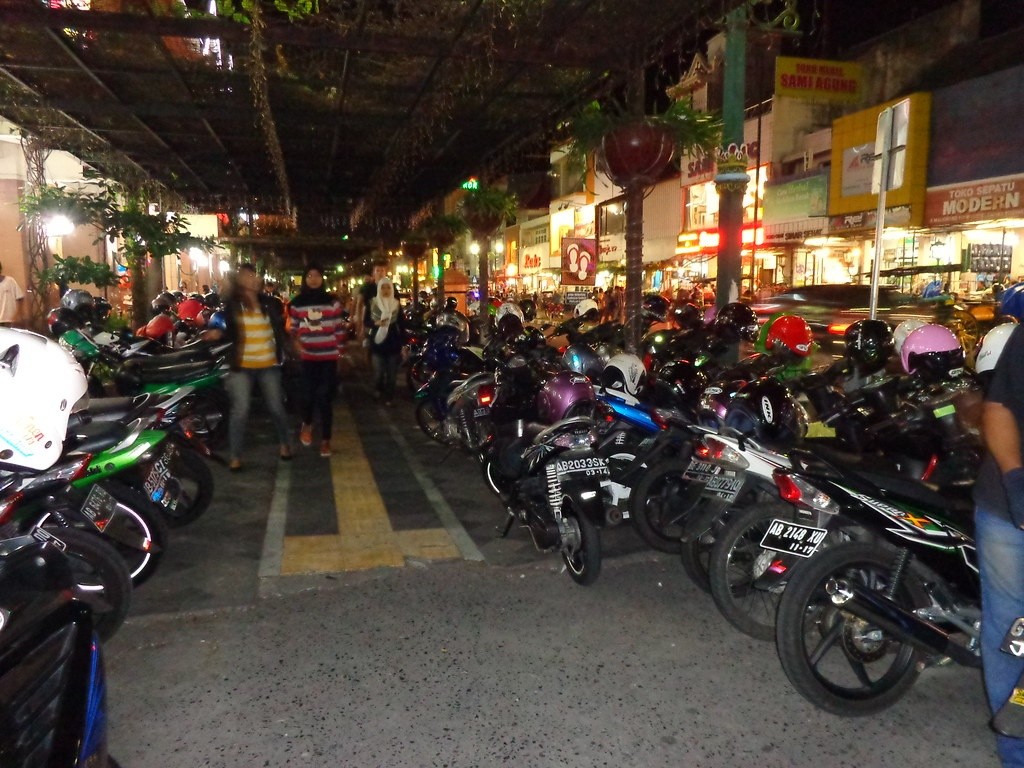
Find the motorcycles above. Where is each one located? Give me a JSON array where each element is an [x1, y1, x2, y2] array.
[[0, 290, 232, 767], [402, 291, 983, 715]]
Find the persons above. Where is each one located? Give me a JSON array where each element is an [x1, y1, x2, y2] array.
[[266, 283, 289, 300], [975, 324, 1024, 768], [346, 260, 408, 409], [690, 282, 716, 300], [340, 284, 351, 301], [588, 288, 605, 309], [0, 261, 24, 327], [467, 291, 479, 305], [420, 287, 437, 309], [496, 288, 539, 310], [202, 284, 212, 293], [287, 263, 345, 457], [600, 286, 624, 326], [224, 263, 294, 472], [179, 281, 189, 296]]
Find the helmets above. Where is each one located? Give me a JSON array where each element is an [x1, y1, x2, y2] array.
[[573, 299, 599, 321], [657, 358, 708, 404], [63, 289, 94, 311], [843, 320, 894, 375], [900, 325, 965, 375], [713, 302, 757, 343], [494, 302, 525, 332], [520, 300, 536, 321], [0, 327, 89, 471], [196, 308, 212, 326], [536, 371, 596, 430], [676, 303, 702, 328], [642, 295, 670, 319], [152, 292, 177, 312], [47, 306, 81, 336], [208, 312, 226, 330], [602, 353, 648, 396], [92, 298, 112, 322], [757, 314, 814, 358]]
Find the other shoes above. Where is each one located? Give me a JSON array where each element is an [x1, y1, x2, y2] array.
[[320, 442, 331, 457], [386, 401, 395, 407], [229, 466, 241, 475], [281, 454, 293, 462], [300, 422, 312, 446], [374, 390, 381, 399]]
